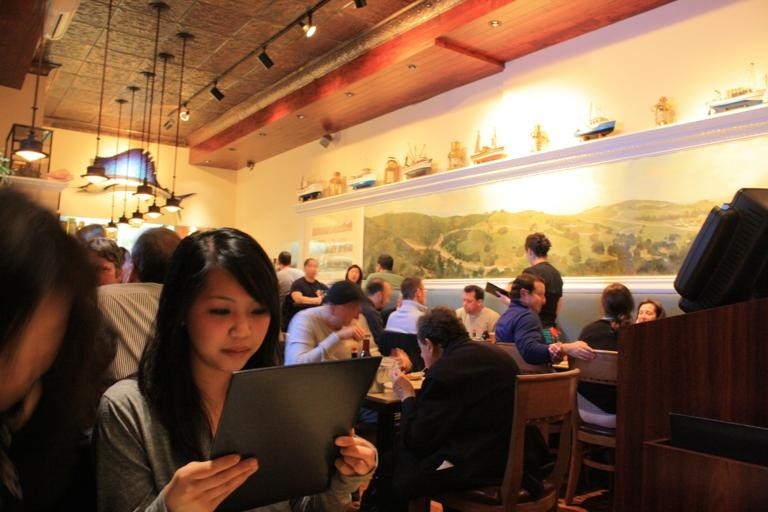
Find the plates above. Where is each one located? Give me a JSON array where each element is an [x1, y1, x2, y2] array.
[[384, 372, 425, 389]]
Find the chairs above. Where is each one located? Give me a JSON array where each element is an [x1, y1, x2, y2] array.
[[408, 368, 581, 512], [565, 349, 620, 506], [496, 342, 563, 443]]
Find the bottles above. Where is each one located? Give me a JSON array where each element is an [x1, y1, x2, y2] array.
[[481, 321, 490, 340], [350, 334, 371, 358]]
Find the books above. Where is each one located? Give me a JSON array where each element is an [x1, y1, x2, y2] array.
[[208, 355, 382, 512]]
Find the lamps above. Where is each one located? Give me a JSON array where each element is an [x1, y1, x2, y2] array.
[[318, 134, 340, 147], [81, 2, 116, 185], [146, 52, 173, 218], [162, 31, 197, 211], [15, 38, 51, 164], [129, 70, 154, 226], [164, 0, 368, 131], [132, 2, 170, 199], [104, 165, 116, 231], [117, 98, 128, 227]]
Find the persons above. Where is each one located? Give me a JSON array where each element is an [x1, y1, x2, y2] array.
[[456, 231, 665, 465], [91, 227, 181, 384], [0, 184, 95, 512], [376, 308, 554, 512], [95, 229, 378, 512], [274, 252, 430, 449]]
